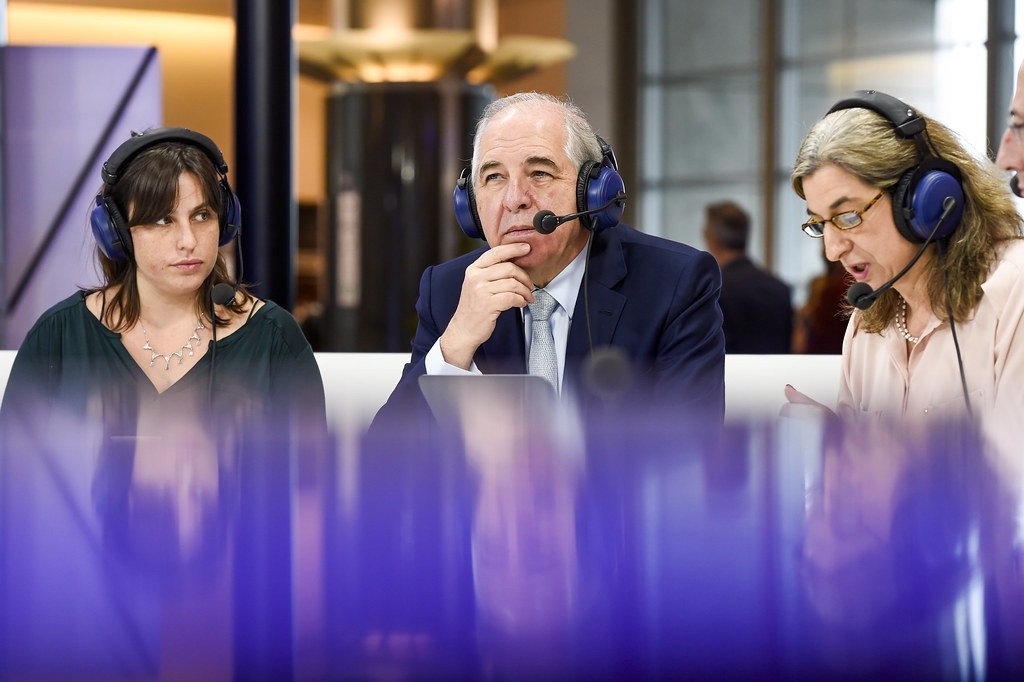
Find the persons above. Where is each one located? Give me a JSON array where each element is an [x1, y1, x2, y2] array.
[[365, 91, 728, 434], [995, 61, 1023, 195], [87, 436, 235, 603], [777, 90, 1023, 531], [372, 436, 648, 679], [793, 240, 856, 356], [786, 420, 972, 629], [701, 200, 798, 354], [0, 127, 327, 434]]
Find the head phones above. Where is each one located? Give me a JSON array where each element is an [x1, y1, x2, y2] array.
[[450, 130, 628, 244], [88, 128, 242, 262], [824, 92, 969, 245]]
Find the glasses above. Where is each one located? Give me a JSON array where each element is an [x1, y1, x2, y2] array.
[[802, 191, 885, 238]]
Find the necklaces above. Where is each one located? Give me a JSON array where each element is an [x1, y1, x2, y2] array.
[[137, 304, 205, 370], [894, 294, 919, 343]]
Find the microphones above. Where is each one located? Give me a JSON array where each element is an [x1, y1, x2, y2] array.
[[846, 195, 953, 310], [532, 191, 628, 235], [211, 223, 243, 305]]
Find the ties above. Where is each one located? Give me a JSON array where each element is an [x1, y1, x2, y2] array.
[[526, 288, 558, 397]]
